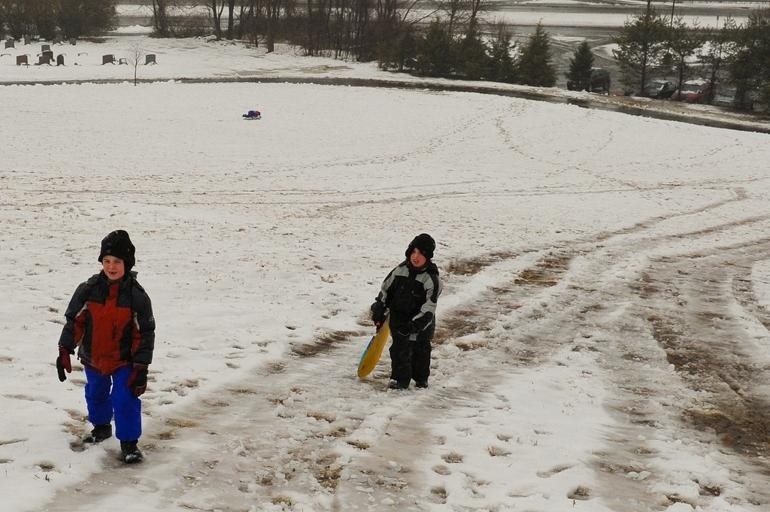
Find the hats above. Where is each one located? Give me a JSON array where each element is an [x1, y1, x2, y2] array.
[[405, 232, 435, 261], [98, 229, 136, 269]]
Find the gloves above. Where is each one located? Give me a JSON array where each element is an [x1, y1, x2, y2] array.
[[371, 308, 386, 329], [56, 346, 72, 382], [126, 364, 148, 397], [399, 320, 416, 335]]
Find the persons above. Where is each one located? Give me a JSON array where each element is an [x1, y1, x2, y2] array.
[[371, 233, 443, 390], [57, 230, 155, 464]]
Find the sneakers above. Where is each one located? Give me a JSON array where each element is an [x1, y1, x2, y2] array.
[[415, 381, 428, 388], [119, 440, 143, 464], [83, 423, 113, 443], [388, 378, 403, 389]]
